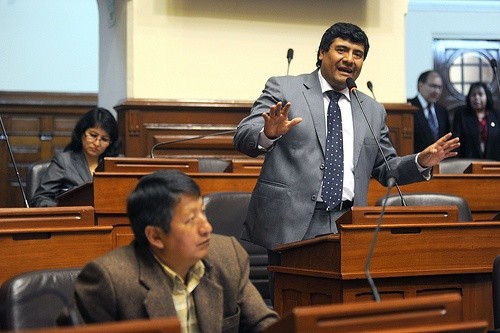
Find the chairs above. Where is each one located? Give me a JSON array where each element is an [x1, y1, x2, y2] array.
[[0, 268, 81, 333], [201, 193, 273, 305], [375, 192, 472, 221]]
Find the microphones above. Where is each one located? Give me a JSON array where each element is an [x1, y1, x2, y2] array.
[[364, 168, 399, 302], [346, 76, 407, 207], [367, 81, 376, 100], [287, 48, 293, 74]]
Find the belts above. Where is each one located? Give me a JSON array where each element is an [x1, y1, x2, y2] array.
[[315, 200, 352, 212]]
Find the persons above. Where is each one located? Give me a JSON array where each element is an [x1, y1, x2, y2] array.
[[30, 108, 118, 207], [55, 170, 282, 333], [454, 82, 500, 163], [234, 22, 460, 306], [407, 71, 451, 153]]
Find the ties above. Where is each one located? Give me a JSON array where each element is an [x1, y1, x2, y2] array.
[[321, 91, 345, 209], [427, 103, 439, 138]]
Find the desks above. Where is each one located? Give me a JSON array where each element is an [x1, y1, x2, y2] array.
[[0, 160, 500, 333]]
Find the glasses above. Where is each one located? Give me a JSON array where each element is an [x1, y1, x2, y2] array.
[[84, 132, 113, 145]]
